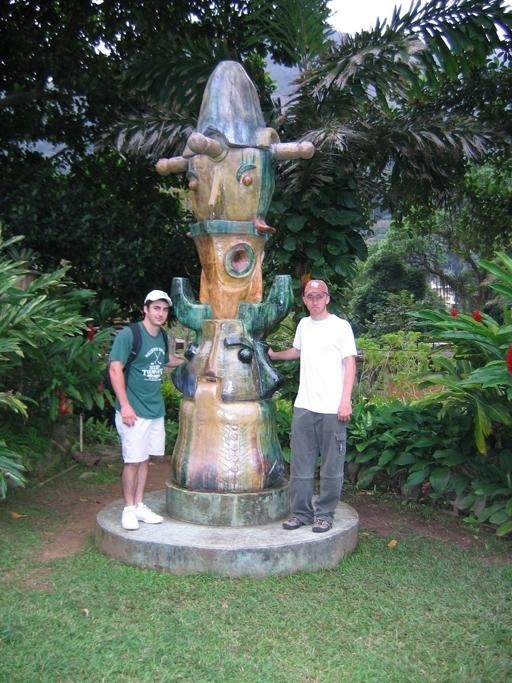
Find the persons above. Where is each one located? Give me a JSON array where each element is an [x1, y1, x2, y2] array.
[[267, 280, 357, 532], [109, 289, 184, 530]]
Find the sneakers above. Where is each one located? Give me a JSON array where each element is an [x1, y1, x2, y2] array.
[[137, 503, 165, 525], [312, 518, 333, 534], [120, 505, 139, 531], [283, 514, 304, 529]]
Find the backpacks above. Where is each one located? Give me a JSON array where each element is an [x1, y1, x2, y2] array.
[[105, 324, 141, 398]]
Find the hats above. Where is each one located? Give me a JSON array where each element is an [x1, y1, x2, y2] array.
[[303, 279, 329, 300], [143, 289, 174, 308]]
